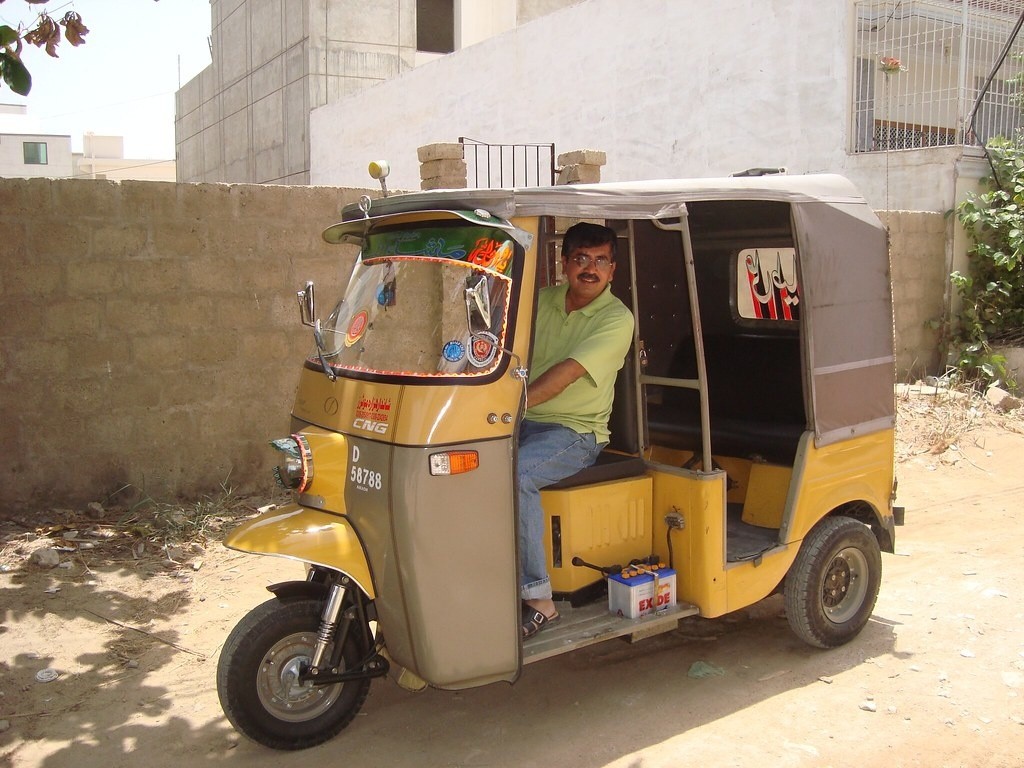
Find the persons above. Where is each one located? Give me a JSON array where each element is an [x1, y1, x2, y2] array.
[[508, 221, 636, 641]]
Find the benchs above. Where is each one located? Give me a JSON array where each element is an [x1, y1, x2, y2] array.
[[538, 454, 656, 608]]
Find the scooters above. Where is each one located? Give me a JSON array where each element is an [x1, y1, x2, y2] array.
[[214, 162, 906, 752]]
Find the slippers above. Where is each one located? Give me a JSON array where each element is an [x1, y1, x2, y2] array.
[[522, 603, 560, 641]]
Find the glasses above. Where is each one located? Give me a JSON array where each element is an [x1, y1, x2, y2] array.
[[568, 256, 612, 269]]
